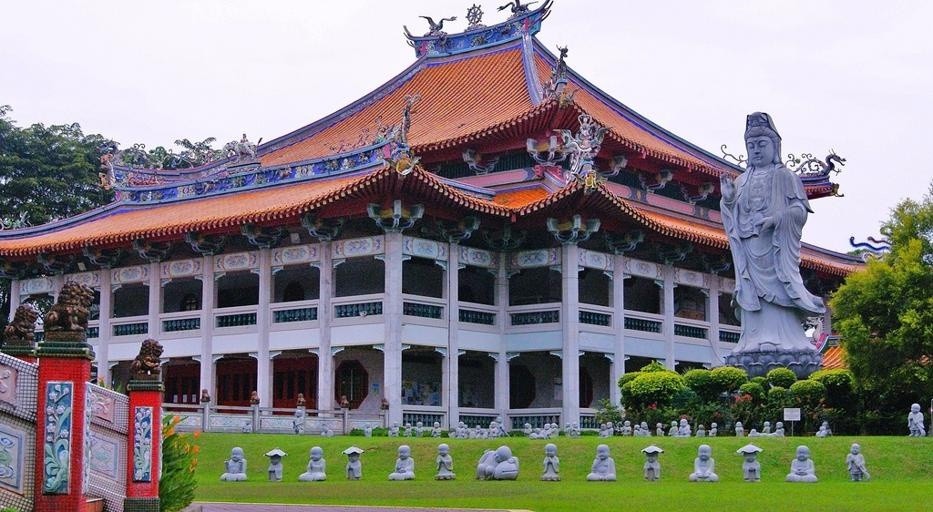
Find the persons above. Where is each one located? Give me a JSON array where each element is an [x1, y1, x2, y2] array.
[[690, 444, 718, 482], [243, 418, 832, 438], [737, 444, 763, 481], [543, 444, 561, 480], [342, 445, 364, 479], [587, 444, 617, 481], [641, 445, 665, 480], [477, 446, 518, 479], [907, 403, 926, 438], [299, 446, 328, 481], [847, 444, 865, 480], [718, 111, 826, 353], [220, 447, 248, 482], [264, 448, 287, 481], [389, 445, 415, 480], [785, 446, 818, 482], [436, 443, 456, 479]]
